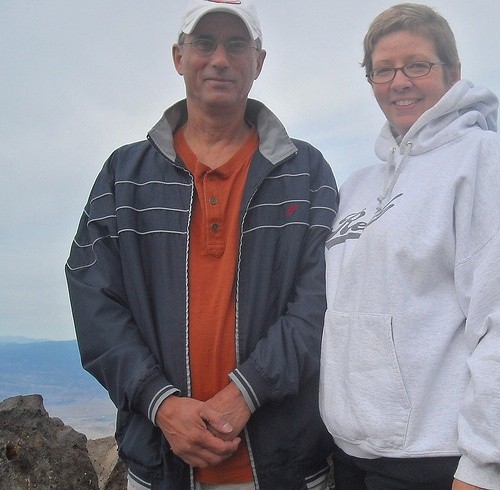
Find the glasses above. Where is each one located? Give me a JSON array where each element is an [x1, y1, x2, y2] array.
[[182, 38, 259, 57], [365, 60, 447, 84]]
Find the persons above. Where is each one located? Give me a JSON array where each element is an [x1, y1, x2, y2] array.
[[64, 0, 343, 490], [313, 3, 500, 489]]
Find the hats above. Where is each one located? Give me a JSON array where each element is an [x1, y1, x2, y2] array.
[[178, 0, 263, 42]]
